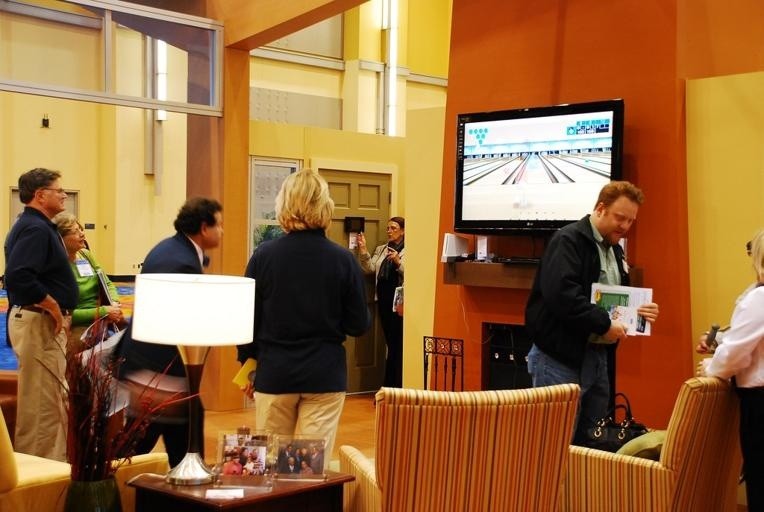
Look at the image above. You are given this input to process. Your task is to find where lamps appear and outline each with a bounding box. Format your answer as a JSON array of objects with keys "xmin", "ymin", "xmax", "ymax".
[{"xmin": 130, "ymin": 273, "xmax": 257, "ymax": 485}]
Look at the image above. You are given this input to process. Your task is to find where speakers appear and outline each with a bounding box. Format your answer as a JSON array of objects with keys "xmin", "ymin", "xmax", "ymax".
[{"xmin": 482, "ymin": 322, "xmax": 535, "ymax": 391}]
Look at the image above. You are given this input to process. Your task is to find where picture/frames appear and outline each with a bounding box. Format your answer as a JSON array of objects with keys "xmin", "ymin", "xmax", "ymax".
[
  {"xmin": 270, "ymin": 433, "xmax": 330, "ymax": 482},
  {"xmin": 215, "ymin": 431, "xmax": 272, "ymax": 484}
]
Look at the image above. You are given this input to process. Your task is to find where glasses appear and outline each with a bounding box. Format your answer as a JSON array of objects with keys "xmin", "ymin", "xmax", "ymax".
[{"xmin": 39, "ymin": 187, "xmax": 67, "ymax": 193}]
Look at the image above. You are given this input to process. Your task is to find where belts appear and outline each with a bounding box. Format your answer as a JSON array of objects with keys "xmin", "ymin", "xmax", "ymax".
[{"xmin": 12, "ymin": 303, "xmax": 74, "ymax": 317}]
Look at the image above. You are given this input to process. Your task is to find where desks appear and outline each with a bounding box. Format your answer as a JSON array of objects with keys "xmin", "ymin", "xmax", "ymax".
[{"xmin": 124, "ymin": 460, "xmax": 356, "ymax": 511}]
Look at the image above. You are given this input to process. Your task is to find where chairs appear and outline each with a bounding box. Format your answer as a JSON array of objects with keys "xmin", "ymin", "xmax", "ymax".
[
  {"xmin": 568, "ymin": 375, "xmax": 744, "ymax": 511},
  {"xmin": 1, "ymin": 403, "xmax": 168, "ymax": 512},
  {"xmin": 339, "ymin": 384, "xmax": 581, "ymax": 512}
]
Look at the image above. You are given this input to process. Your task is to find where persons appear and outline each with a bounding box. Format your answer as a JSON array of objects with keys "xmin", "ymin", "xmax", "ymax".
[
  {"xmin": 278, "ymin": 443, "xmax": 324, "ymax": 474},
  {"xmin": 223, "ymin": 427, "xmax": 268, "ymax": 476},
  {"xmin": 696, "ymin": 231, "xmax": 763, "ymax": 512},
  {"xmin": 3, "ymin": 168, "xmax": 122, "ymax": 463},
  {"xmin": 525, "ymin": 179, "xmax": 658, "ymax": 442},
  {"xmin": 357, "ymin": 217, "xmax": 405, "ymax": 388},
  {"xmin": 114, "ymin": 197, "xmax": 224, "ymax": 469},
  {"xmin": 234, "ymin": 167, "xmax": 373, "ymax": 456}
]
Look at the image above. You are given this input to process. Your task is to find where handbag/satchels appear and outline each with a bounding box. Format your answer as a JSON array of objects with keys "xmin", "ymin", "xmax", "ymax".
[{"xmin": 584, "ymin": 414, "xmax": 650, "ymax": 453}]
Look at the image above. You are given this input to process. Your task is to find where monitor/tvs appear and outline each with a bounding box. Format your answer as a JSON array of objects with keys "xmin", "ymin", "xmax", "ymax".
[{"xmin": 454, "ymin": 99, "xmax": 625, "ymax": 264}]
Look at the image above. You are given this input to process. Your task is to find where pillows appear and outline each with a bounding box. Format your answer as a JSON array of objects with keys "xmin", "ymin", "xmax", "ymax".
[{"xmin": 615, "ymin": 433, "xmax": 665, "ymax": 461}]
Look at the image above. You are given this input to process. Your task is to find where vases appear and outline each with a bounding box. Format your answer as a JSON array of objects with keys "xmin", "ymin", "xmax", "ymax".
[{"xmin": 62, "ymin": 475, "xmax": 123, "ymax": 512}]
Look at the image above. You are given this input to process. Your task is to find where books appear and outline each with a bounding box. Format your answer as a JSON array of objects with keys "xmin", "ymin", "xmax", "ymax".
[
  {"xmin": 590, "ymin": 282, "xmax": 653, "ymax": 337},
  {"xmin": 231, "ymin": 357, "xmax": 258, "ymax": 389},
  {"xmin": 79, "ymin": 313, "xmax": 118, "ymax": 349}
]
